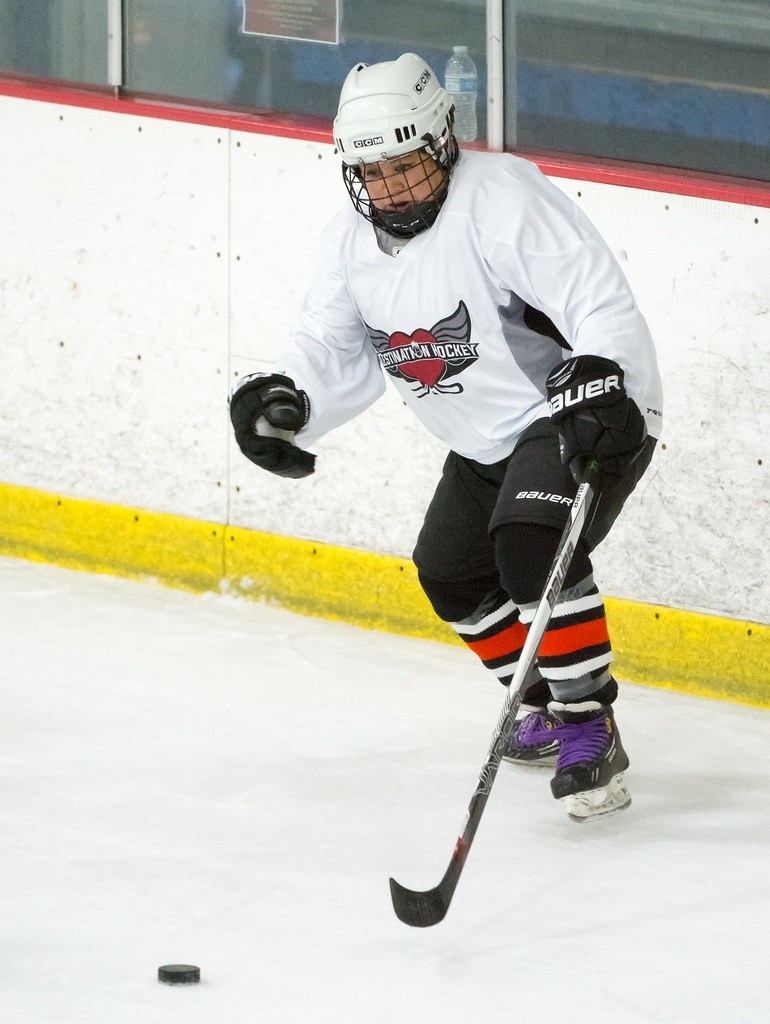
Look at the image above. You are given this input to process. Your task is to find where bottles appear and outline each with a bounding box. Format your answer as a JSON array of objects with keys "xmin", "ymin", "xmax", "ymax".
[{"xmin": 445, "ymin": 45, "xmax": 478, "ymax": 144}]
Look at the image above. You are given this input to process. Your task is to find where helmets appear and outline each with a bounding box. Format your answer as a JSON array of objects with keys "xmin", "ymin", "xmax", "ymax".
[{"xmin": 331, "ymin": 53, "xmax": 455, "ymax": 239}]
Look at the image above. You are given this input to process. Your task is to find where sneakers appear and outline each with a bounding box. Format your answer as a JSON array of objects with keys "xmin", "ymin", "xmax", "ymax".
[
  {"xmin": 500, "ymin": 710, "xmax": 564, "ymax": 767},
  {"xmin": 547, "ymin": 698, "xmax": 635, "ymax": 825}
]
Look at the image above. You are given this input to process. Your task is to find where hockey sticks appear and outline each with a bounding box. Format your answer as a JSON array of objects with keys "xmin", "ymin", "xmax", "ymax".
[{"xmin": 390, "ymin": 455, "xmax": 603, "ymax": 929}]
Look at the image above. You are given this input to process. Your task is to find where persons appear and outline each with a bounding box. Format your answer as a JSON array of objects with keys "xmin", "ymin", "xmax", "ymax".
[{"xmin": 228, "ymin": 52, "xmax": 662, "ymax": 825}]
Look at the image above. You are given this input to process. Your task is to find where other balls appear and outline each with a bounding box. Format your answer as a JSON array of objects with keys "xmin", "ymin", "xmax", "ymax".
[{"xmin": 158, "ymin": 963, "xmax": 201, "ymax": 985}]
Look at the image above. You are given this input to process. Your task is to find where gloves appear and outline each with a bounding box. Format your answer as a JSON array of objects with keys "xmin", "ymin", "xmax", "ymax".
[
  {"xmin": 545, "ymin": 355, "xmax": 648, "ymax": 490},
  {"xmin": 224, "ymin": 370, "xmax": 318, "ymax": 479}
]
